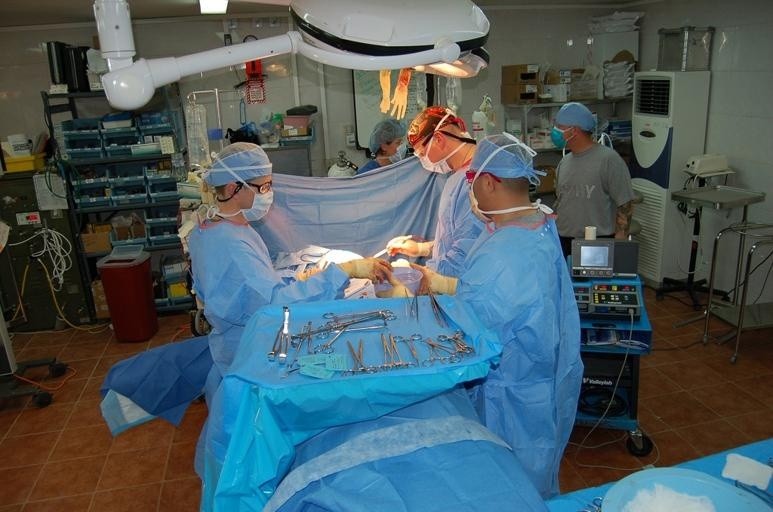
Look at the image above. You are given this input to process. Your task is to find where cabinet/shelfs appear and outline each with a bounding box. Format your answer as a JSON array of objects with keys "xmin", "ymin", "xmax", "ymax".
[
  {"xmin": 500, "ymin": 93, "xmax": 631, "ymax": 216},
  {"xmin": 36, "ymin": 73, "xmax": 205, "ymax": 325}
]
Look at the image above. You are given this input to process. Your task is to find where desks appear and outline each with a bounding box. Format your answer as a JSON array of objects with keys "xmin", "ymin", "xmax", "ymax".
[{"xmin": 541, "ymin": 426, "xmax": 772, "ymax": 511}]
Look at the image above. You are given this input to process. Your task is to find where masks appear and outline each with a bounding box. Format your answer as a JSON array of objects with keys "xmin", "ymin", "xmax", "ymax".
[
  {"xmin": 418, "ymin": 157, "xmax": 454, "ymax": 175},
  {"xmin": 467, "ymin": 189, "xmax": 492, "ymax": 225},
  {"xmin": 239, "ymin": 191, "xmax": 273, "ymax": 223},
  {"xmin": 388, "ymin": 143, "xmax": 408, "ymax": 166},
  {"xmin": 549, "ymin": 127, "xmax": 566, "ymax": 150}
]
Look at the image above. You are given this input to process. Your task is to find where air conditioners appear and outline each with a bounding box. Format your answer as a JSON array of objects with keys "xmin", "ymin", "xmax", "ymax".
[{"xmin": 623, "ymin": 69, "xmax": 712, "ymax": 296}]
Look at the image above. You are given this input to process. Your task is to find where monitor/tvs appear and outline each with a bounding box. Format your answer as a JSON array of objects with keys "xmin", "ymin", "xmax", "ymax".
[{"xmin": 263, "ymin": 145, "xmax": 313, "ymax": 178}]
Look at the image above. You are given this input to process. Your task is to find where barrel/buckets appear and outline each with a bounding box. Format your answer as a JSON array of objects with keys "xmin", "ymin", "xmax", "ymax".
[{"xmin": 472, "ymin": 109, "xmax": 489, "ymax": 140}]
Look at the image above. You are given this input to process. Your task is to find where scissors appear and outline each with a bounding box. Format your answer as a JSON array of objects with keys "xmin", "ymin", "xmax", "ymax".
[
  {"xmin": 287, "ymin": 310, "xmax": 475, "ymax": 376},
  {"xmin": 584, "ymin": 497, "xmax": 603, "ymax": 512}
]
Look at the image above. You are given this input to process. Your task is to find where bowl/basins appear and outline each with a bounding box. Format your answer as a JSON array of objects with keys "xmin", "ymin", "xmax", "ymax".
[{"xmin": 375, "ymin": 268, "xmax": 422, "ymax": 298}]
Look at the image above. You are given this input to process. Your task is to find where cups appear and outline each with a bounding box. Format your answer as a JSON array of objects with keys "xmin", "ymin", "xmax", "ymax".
[{"xmin": 583, "ymin": 225, "xmax": 595, "ymax": 240}]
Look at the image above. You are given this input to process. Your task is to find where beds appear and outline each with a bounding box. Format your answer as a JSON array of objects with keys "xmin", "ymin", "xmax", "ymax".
[{"xmin": 184, "ymin": 245, "xmax": 549, "ymax": 512}]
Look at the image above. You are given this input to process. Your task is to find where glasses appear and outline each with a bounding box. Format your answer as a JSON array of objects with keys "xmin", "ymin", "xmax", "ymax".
[
  {"xmin": 243, "ymin": 179, "xmax": 273, "ymax": 193},
  {"xmin": 412, "ymin": 134, "xmax": 436, "ymax": 157},
  {"xmin": 464, "ymin": 169, "xmax": 500, "ymax": 185}
]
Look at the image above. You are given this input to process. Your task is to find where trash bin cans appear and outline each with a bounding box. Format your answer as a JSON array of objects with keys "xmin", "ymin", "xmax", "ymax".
[{"xmin": 96, "ymin": 245, "xmax": 160, "ymax": 343}]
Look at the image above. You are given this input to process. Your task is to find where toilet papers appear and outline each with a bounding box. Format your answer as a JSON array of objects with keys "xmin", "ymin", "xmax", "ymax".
[{"xmin": 584, "ymin": 226, "xmax": 597, "ymax": 240}]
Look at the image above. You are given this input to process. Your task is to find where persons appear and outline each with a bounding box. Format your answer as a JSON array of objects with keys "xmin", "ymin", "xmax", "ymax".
[
  {"xmin": 369, "ymin": 131, "xmax": 585, "ymax": 500},
  {"xmin": 383, "ymin": 105, "xmax": 492, "ymax": 281},
  {"xmin": 548, "ymin": 102, "xmax": 636, "ymax": 261},
  {"xmin": 353, "ymin": 118, "xmax": 407, "ymax": 176},
  {"xmin": 188, "ymin": 138, "xmax": 394, "ymax": 378}
]
